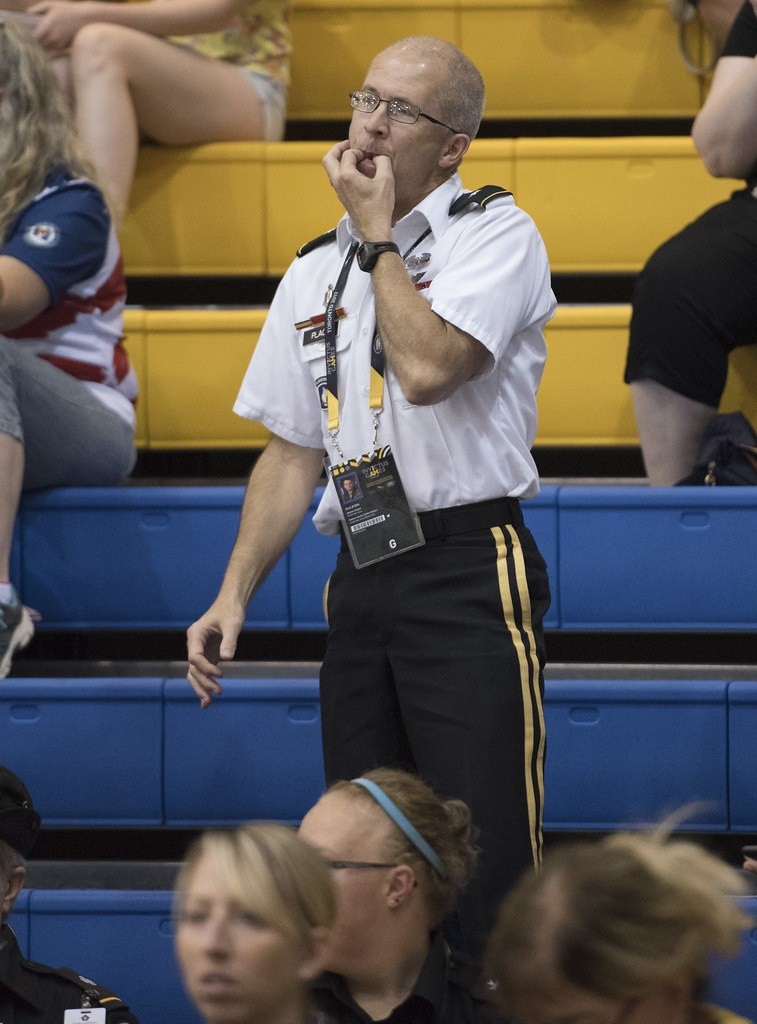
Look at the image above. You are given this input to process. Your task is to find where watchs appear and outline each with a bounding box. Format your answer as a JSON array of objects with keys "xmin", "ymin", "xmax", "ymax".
[{"xmin": 357, "ymin": 240, "xmax": 401, "ymax": 272}]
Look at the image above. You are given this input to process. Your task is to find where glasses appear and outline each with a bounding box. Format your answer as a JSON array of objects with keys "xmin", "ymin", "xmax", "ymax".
[{"xmin": 349, "ymin": 89, "xmax": 459, "ymax": 134}]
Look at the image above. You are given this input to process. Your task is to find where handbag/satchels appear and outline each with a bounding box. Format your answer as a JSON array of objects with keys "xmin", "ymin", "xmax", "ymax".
[{"xmin": 672, "ymin": 410, "xmax": 757, "ymax": 487}]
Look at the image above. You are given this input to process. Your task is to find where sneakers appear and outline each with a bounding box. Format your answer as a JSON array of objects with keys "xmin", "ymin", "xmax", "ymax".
[{"xmin": 0, "ymin": 601, "xmax": 34, "ymax": 679}]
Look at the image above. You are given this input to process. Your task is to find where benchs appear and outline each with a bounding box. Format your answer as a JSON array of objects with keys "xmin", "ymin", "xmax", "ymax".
[{"xmin": 1, "ymin": 0, "xmax": 756, "ymax": 1024}]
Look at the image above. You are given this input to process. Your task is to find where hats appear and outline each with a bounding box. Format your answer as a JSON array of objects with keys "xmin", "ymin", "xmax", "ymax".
[{"xmin": 0, "ymin": 765, "xmax": 41, "ymax": 862}]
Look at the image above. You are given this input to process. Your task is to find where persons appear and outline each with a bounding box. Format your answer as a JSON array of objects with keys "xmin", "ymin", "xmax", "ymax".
[
  {"xmin": 1, "ymin": 768, "xmax": 141, "ymax": 1024},
  {"xmin": 174, "ymin": 768, "xmax": 757, "ymax": 1024},
  {"xmin": 623, "ymin": 0, "xmax": 757, "ymax": 488},
  {"xmin": 27, "ymin": 1, "xmax": 289, "ymax": 225},
  {"xmin": 0, "ymin": 17, "xmax": 134, "ymax": 678},
  {"xmin": 188, "ymin": 34, "xmax": 550, "ymax": 960}
]
